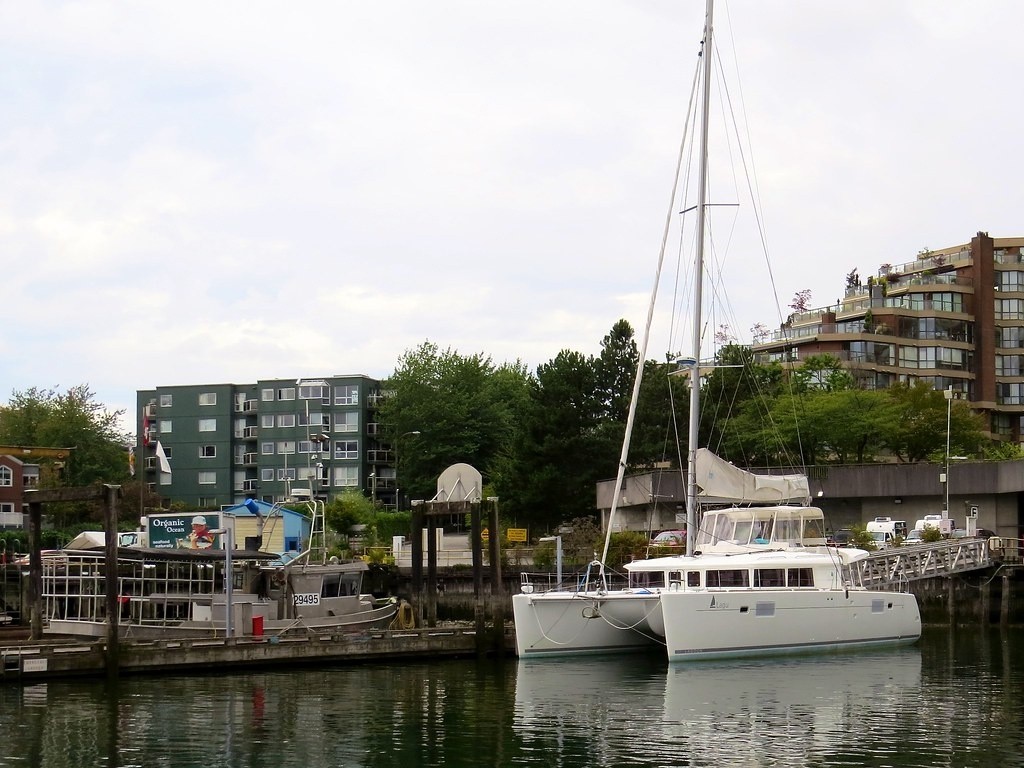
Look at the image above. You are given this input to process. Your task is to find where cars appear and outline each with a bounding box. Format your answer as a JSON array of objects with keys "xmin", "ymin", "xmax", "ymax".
[
  {"xmin": 653, "ymin": 530, "xmax": 686, "ymax": 552},
  {"xmin": 857, "ymin": 530, "xmax": 894, "ymax": 552},
  {"xmin": 832, "ymin": 528, "xmax": 859, "ymax": 550},
  {"xmin": 900, "ymin": 528, "xmax": 926, "ymax": 546}
]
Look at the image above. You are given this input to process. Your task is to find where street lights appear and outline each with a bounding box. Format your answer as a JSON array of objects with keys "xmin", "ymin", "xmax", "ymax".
[
  {"xmin": 395, "ymin": 431, "xmax": 421, "ymax": 489},
  {"xmin": 209, "ymin": 528, "xmax": 235, "ymax": 638}
]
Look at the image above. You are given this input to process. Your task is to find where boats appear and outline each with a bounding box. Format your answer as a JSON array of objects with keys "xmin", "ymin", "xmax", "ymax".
[{"xmin": 12, "ymin": 547, "xmax": 402, "ymax": 651}]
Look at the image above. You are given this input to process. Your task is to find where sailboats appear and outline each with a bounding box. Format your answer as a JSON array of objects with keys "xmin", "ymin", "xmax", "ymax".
[{"xmin": 510, "ymin": 1, "xmax": 927, "ymax": 663}]
